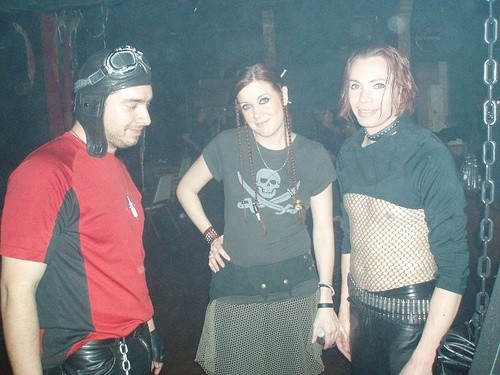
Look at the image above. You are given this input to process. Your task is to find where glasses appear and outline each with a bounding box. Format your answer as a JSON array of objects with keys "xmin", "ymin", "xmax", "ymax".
[{"xmin": 103, "ymin": 46, "xmax": 152, "ymax": 77}]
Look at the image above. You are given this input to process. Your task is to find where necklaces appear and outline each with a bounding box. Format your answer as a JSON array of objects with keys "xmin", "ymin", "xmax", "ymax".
[
  {"xmin": 71, "ymin": 129, "xmax": 138, "ymax": 221},
  {"xmin": 253, "ymin": 131, "xmax": 290, "ymax": 172}
]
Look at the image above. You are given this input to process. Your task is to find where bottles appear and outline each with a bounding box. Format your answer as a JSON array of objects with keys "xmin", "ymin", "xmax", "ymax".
[{"xmin": 319, "ymin": 331, "xmax": 338, "ymax": 350}]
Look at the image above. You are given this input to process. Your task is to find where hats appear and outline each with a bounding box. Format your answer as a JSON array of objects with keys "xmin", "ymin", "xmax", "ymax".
[{"xmin": 76, "ymin": 49, "xmax": 152, "ymax": 158}]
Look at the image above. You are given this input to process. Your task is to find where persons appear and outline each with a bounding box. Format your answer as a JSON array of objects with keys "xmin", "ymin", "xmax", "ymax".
[
  {"xmin": 335, "ymin": 45, "xmax": 470, "ymax": 375},
  {"xmin": 0, "ymin": 45, "xmax": 165, "ymax": 375},
  {"xmin": 175, "ymin": 63, "xmax": 348, "ymax": 375}
]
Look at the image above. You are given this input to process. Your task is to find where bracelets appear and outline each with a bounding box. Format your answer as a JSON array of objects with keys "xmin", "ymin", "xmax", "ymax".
[
  {"xmin": 202, "ymin": 226, "xmax": 219, "ymax": 245},
  {"xmin": 317, "ymin": 282, "xmax": 335, "ymax": 296},
  {"xmin": 317, "ymin": 302, "xmax": 335, "ymax": 309}
]
